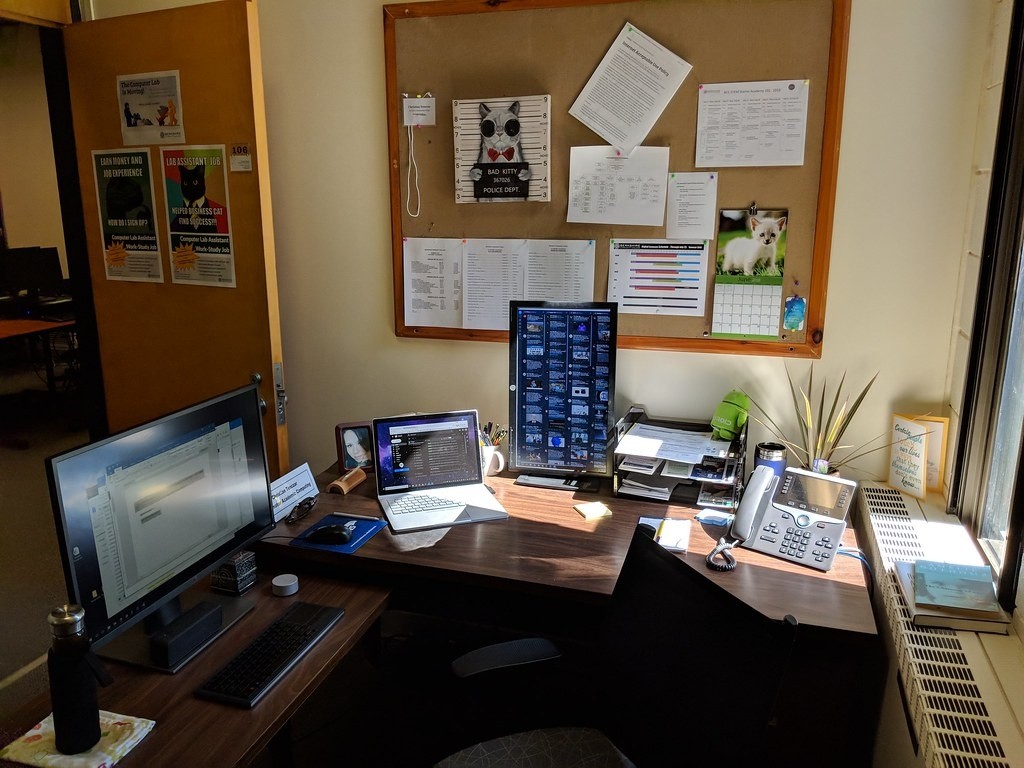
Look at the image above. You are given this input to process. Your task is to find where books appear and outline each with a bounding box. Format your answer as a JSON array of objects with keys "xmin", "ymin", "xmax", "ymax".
[
  {"xmin": 618, "ymin": 472, "xmax": 679, "ymax": 500},
  {"xmin": 574, "ymin": 501, "xmax": 612, "ymax": 519},
  {"xmin": 638, "ymin": 517, "xmax": 691, "ymax": 554},
  {"xmin": 893, "ymin": 560, "xmax": 1012, "ymax": 635}
]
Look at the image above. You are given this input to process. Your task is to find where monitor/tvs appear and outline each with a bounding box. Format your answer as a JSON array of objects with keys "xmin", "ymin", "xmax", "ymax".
[{"xmin": 45, "ymin": 383, "xmax": 277, "ymax": 674}]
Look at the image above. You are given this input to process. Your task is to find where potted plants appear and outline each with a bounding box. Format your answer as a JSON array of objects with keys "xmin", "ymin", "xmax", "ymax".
[{"xmin": 736, "ymin": 360, "xmax": 936, "ymax": 479}]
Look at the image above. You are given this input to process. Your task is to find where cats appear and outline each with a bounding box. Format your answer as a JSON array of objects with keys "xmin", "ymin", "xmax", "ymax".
[
  {"xmin": 722, "ymin": 217, "xmax": 785, "ymax": 276},
  {"xmin": 169, "ymin": 159, "xmax": 228, "ymax": 233},
  {"xmin": 469, "ymin": 101, "xmax": 532, "ymax": 202}
]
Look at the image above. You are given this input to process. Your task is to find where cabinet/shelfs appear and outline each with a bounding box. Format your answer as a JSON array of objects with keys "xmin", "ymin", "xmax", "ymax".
[{"xmin": 606, "ymin": 406, "xmax": 749, "ymax": 514}]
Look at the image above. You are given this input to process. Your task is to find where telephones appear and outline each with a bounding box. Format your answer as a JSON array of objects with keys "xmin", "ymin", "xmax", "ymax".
[{"xmin": 730, "ymin": 464, "xmax": 857, "ymax": 571}]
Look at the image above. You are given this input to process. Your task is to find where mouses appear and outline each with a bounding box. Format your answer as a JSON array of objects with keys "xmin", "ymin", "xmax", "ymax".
[{"xmin": 304, "ymin": 524, "xmax": 352, "ymax": 545}]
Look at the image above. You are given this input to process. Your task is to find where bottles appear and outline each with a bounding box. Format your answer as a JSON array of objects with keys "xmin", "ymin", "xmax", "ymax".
[{"xmin": 47, "ymin": 604, "xmax": 102, "ymax": 754}]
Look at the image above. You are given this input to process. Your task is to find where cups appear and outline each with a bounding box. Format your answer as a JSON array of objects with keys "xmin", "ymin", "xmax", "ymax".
[
  {"xmin": 755, "ymin": 442, "xmax": 787, "ymax": 480},
  {"xmin": 482, "ymin": 444, "xmax": 505, "ymax": 476}
]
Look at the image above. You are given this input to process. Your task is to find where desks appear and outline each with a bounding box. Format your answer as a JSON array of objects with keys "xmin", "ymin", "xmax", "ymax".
[{"xmin": 0, "ymin": 319, "xmax": 873, "ymax": 768}]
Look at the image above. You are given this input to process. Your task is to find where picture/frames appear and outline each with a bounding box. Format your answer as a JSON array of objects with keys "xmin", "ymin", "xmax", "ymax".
[{"xmin": 335, "ymin": 420, "xmax": 375, "ymax": 472}]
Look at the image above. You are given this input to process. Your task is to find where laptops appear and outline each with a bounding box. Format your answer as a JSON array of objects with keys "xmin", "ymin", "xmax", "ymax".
[{"xmin": 372, "ymin": 410, "xmax": 508, "ymax": 533}]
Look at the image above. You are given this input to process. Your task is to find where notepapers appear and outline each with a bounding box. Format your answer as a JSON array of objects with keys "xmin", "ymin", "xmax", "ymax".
[{"xmin": 574, "ymin": 501, "xmax": 612, "ymax": 519}]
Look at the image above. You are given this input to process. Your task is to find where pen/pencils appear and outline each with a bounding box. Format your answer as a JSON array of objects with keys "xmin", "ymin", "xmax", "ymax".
[
  {"xmin": 479, "ymin": 421, "xmax": 508, "ymax": 446},
  {"xmin": 332, "ymin": 511, "xmax": 380, "ymax": 520},
  {"xmin": 655, "ymin": 520, "xmax": 667, "ymax": 543}
]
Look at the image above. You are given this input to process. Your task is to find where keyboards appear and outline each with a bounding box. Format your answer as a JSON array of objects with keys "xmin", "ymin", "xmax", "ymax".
[{"xmin": 192, "ymin": 601, "xmax": 345, "ymax": 710}]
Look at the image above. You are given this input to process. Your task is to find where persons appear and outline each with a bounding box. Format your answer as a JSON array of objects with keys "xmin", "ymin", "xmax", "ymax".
[{"xmin": 344, "ymin": 429, "xmax": 372, "ymax": 469}]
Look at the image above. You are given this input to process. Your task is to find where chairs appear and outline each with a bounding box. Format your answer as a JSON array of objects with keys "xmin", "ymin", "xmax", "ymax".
[{"xmin": 429, "ymin": 636, "xmax": 637, "ymax": 768}]
